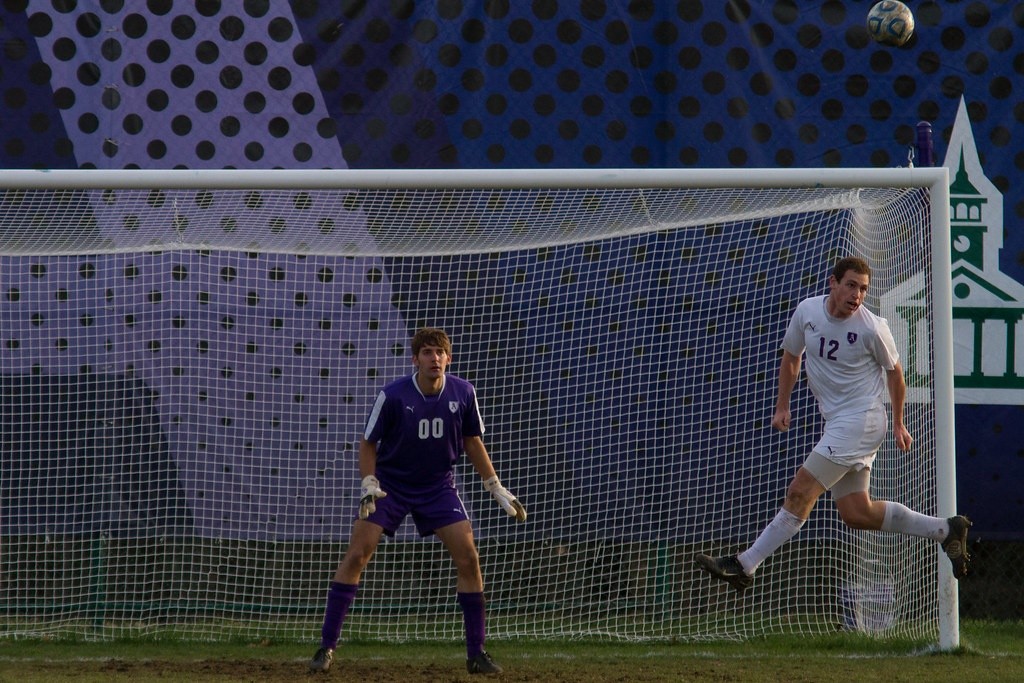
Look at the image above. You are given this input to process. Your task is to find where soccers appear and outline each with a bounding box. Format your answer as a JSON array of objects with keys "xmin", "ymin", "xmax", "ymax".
[{"xmin": 865, "ymin": 0, "xmax": 914, "ymax": 49}]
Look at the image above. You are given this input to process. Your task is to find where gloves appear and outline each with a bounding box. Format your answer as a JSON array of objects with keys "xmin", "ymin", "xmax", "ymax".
[
  {"xmin": 359, "ymin": 475, "xmax": 387, "ymax": 519},
  {"xmin": 484, "ymin": 475, "xmax": 526, "ymax": 521}
]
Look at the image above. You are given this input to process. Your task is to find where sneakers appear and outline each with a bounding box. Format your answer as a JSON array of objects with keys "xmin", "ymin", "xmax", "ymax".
[
  {"xmin": 310, "ymin": 647, "xmax": 333, "ymax": 671},
  {"xmin": 695, "ymin": 553, "xmax": 754, "ymax": 591},
  {"xmin": 941, "ymin": 515, "xmax": 972, "ymax": 580},
  {"xmin": 466, "ymin": 651, "xmax": 503, "ymax": 674}
]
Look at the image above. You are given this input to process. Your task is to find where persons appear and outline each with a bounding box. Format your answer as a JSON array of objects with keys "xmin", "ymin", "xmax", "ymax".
[
  {"xmin": 308, "ymin": 328, "xmax": 526, "ymax": 675},
  {"xmin": 695, "ymin": 257, "xmax": 972, "ymax": 592}
]
